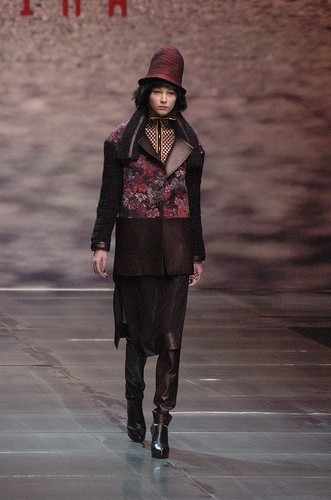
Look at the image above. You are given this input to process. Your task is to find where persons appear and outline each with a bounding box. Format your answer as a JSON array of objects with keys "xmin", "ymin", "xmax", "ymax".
[{"xmin": 91, "ymin": 45, "xmax": 206, "ymax": 458}]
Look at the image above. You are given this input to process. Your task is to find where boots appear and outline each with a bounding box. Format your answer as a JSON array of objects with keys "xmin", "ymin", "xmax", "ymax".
[
  {"xmin": 126, "ymin": 398, "xmax": 146, "ymax": 443},
  {"xmin": 149, "ymin": 408, "xmax": 172, "ymax": 459}
]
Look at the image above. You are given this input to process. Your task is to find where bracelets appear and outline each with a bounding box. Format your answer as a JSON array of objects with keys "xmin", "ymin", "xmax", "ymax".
[
  {"xmin": 194, "ymin": 254, "xmax": 205, "ymax": 261},
  {"xmin": 91, "ymin": 241, "xmax": 110, "ymax": 252}
]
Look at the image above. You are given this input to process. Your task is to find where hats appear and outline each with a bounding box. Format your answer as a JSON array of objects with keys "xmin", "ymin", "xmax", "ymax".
[{"xmin": 138, "ymin": 45, "xmax": 188, "ymax": 94}]
